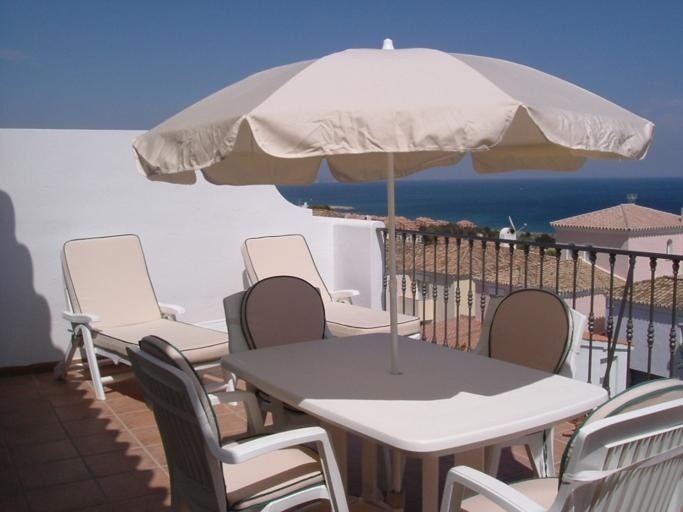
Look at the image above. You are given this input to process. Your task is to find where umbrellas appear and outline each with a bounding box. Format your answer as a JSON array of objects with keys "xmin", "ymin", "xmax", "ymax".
[{"xmin": 129, "ymin": 40, "xmax": 655, "ymax": 495}]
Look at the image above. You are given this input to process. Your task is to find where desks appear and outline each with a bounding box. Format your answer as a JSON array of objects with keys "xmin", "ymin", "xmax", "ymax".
[{"xmin": 218, "ymin": 331, "xmax": 611, "ymax": 512}]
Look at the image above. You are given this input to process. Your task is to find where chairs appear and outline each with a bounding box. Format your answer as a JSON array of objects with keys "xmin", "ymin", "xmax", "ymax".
[
  {"xmin": 437, "ymin": 375, "xmax": 683, "ymax": 511},
  {"xmin": 56, "ymin": 234, "xmax": 237, "ymax": 405},
  {"xmin": 427, "ymin": 287, "xmax": 588, "ymax": 481},
  {"xmin": 242, "ymin": 235, "xmax": 424, "ymax": 345},
  {"xmin": 240, "ymin": 274, "xmax": 343, "ymax": 436},
  {"xmin": 124, "ymin": 333, "xmax": 352, "ymax": 511}
]
